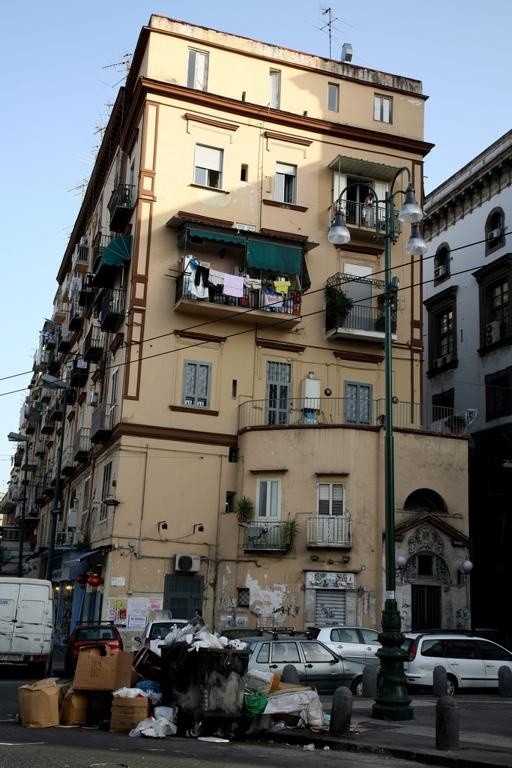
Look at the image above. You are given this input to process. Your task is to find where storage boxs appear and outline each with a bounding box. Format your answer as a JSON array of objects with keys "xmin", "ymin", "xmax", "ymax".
[{"xmin": 18, "ymin": 643, "xmax": 151, "ymax": 734}]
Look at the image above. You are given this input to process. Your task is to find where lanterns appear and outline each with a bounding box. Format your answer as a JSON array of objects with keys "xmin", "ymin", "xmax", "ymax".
[
  {"xmin": 87, "ymin": 572, "xmax": 103, "ymax": 592},
  {"xmin": 76, "ymin": 575, "xmax": 87, "ymax": 584}
]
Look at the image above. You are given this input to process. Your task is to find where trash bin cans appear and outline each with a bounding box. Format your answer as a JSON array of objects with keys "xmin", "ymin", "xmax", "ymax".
[{"xmin": 158, "ymin": 644, "xmax": 254, "ymax": 739}]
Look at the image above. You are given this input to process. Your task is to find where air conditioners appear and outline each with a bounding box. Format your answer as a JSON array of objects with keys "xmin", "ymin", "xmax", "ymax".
[
  {"xmin": 485, "ymin": 227, "xmax": 502, "ymax": 244},
  {"xmin": 484, "ymin": 319, "xmax": 501, "ymax": 346},
  {"xmin": 432, "ymin": 265, "xmax": 449, "ymax": 278},
  {"xmin": 173, "ymin": 553, "xmax": 202, "ymax": 574},
  {"xmin": 430, "ymin": 353, "xmax": 454, "ymax": 370}
]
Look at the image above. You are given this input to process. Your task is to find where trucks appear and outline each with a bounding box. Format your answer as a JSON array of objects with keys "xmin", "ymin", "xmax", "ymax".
[{"xmin": 0, "ymin": 576, "xmax": 57, "ymax": 680}]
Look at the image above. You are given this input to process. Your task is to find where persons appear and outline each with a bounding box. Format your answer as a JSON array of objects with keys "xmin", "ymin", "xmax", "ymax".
[
  {"xmin": 362, "ymin": 192, "xmax": 375, "ymax": 226},
  {"xmin": 192, "ymin": 609, "xmax": 205, "ymax": 628}
]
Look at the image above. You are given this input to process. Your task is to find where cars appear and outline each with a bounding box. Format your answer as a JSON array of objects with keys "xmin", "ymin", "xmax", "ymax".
[
  {"xmin": 305, "ymin": 624, "xmax": 384, "ymax": 675},
  {"xmin": 134, "ymin": 618, "xmax": 189, "ymax": 658}
]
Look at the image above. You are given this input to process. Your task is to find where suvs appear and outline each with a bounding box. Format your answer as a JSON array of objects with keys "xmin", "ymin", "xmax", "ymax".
[
  {"xmin": 63, "ymin": 619, "xmax": 124, "ymax": 675},
  {"xmin": 237, "ymin": 626, "xmax": 381, "ymax": 697}
]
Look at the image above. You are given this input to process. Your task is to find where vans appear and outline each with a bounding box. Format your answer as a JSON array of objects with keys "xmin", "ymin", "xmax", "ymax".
[{"xmin": 401, "ymin": 630, "xmax": 512, "ymax": 698}]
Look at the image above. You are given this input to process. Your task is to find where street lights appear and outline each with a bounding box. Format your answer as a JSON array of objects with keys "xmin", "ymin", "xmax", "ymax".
[{"xmin": 325, "ymin": 165, "xmax": 430, "ymax": 722}]
